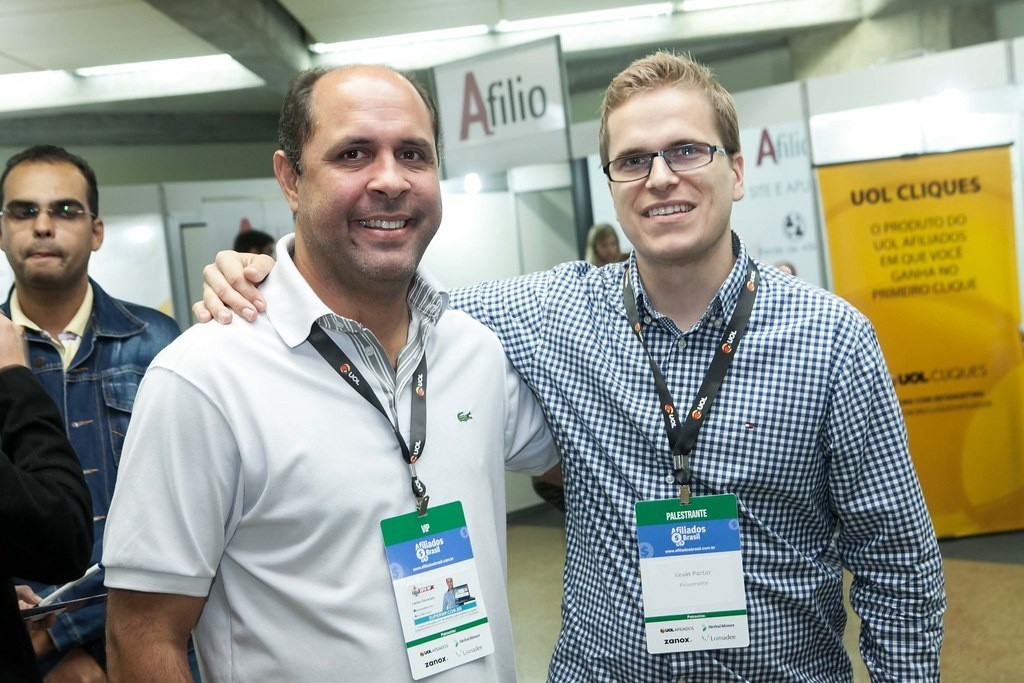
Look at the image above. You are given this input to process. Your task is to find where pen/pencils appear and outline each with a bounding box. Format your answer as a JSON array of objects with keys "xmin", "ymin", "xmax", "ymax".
[{"xmin": 31, "ymin": 562, "xmax": 105, "ymax": 607}]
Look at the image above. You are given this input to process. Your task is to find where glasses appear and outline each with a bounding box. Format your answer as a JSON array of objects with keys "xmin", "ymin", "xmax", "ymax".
[
  {"xmin": 603, "ymin": 142, "xmax": 735, "ymax": 183},
  {"xmin": 0, "ymin": 203, "xmax": 98, "ymax": 219}
]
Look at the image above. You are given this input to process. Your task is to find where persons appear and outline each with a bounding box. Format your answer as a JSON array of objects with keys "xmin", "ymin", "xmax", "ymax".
[
  {"xmin": 586, "ymin": 224, "xmax": 632, "ymax": 269},
  {"xmin": 193, "ymin": 52, "xmax": 947, "ymax": 683},
  {"xmin": 443, "ymin": 578, "xmax": 463, "ymax": 611},
  {"xmin": 100, "ymin": 61, "xmax": 563, "ymax": 683},
  {"xmin": 234, "ymin": 230, "xmax": 275, "ymax": 261},
  {"xmin": 0, "ymin": 145, "xmax": 183, "ymax": 683}
]
